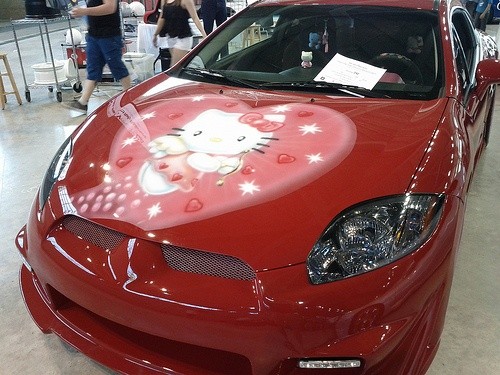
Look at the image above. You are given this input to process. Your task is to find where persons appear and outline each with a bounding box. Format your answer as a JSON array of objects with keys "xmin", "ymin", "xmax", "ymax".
[
  {"xmin": 152, "ymin": 0, "xmax": 207, "ymax": 68},
  {"xmin": 198, "ymin": 0, "xmax": 229, "ymax": 60},
  {"xmin": 61, "ymin": 0, "xmax": 131, "ymax": 114},
  {"xmin": 474, "ymin": 0, "xmax": 493, "ymax": 32}
]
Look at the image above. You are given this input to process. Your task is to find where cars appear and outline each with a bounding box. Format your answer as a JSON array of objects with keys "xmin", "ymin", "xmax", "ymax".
[{"xmin": 15, "ymin": 0, "xmax": 500, "ymax": 375}]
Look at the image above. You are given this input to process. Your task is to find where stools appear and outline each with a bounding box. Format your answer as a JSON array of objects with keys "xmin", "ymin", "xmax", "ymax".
[
  {"xmin": 0, "ymin": 51, "xmax": 23, "ymax": 110},
  {"xmin": 242, "ymin": 24, "xmax": 262, "ymax": 48}
]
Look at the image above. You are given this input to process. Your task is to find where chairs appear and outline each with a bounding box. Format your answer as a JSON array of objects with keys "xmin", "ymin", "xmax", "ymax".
[
  {"xmin": 371, "ymin": 22, "xmax": 430, "ymax": 80},
  {"xmin": 282, "ymin": 29, "xmax": 331, "ymax": 70},
  {"xmin": 254, "ymin": 15, "xmax": 274, "ymax": 39}
]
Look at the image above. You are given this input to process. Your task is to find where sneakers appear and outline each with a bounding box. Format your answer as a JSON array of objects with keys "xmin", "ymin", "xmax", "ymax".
[{"xmin": 61, "ymin": 101, "xmax": 87, "ymax": 113}]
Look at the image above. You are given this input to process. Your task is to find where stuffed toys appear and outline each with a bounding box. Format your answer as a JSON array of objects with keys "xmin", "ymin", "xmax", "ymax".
[
  {"xmin": 301, "ymin": 51, "xmax": 312, "ymax": 68},
  {"xmin": 309, "ymin": 32, "xmax": 321, "ymax": 50},
  {"xmin": 407, "ymin": 36, "xmax": 424, "ymax": 53}
]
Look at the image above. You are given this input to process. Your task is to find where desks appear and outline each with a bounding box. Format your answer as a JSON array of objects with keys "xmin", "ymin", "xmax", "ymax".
[{"xmin": 136, "ymin": 20, "xmax": 217, "ymax": 54}]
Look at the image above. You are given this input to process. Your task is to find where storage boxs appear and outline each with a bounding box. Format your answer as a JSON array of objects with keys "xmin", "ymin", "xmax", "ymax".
[{"xmin": 121, "ymin": 54, "xmax": 155, "ymax": 84}]
